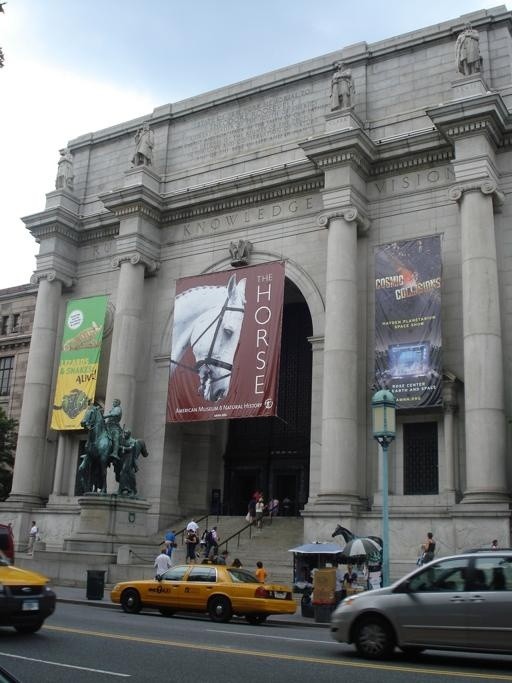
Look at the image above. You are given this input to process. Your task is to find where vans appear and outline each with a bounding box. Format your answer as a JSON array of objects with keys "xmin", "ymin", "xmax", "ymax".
[{"xmin": 0, "ymin": 524, "xmax": 16, "ymax": 565}]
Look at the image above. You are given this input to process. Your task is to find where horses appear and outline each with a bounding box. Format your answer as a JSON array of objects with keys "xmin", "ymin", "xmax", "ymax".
[
  {"xmin": 331, "ymin": 524, "xmax": 383, "ymax": 549},
  {"xmin": 78, "ymin": 403, "xmax": 149, "ymax": 493},
  {"xmin": 170, "ymin": 272, "xmax": 247, "ymax": 403}
]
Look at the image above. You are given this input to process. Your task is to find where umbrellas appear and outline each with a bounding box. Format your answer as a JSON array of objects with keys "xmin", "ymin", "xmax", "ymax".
[{"xmin": 342, "ymin": 537, "xmax": 383, "ymax": 571}]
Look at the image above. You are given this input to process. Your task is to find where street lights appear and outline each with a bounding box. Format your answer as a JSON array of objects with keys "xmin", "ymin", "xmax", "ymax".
[{"xmin": 373, "ymin": 390, "xmax": 398, "ymax": 588}]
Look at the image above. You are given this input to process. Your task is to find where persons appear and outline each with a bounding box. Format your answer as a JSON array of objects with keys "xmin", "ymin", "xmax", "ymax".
[
  {"xmin": 491, "ymin": 539, "xmax": 498, "ymax": 549},
  {"xmin": 330, "ymin": 559, "xmax": 345, "ymax": 603},
  {"xmin": 454, "ymin": 20, "xmax": 485, "ymax": 76},
  {"xmin": 55, "ymin": 147, "xmax": 76, "ymax": 192},
  {"xmin": 255, "ymin": 561, "xmax": 268, "ymax": 583},
  {"xmin": 117, "ymin": 428, "xmax": 138, "ymax": 495},
  {"xmin": 343, "ymin": 564, "xmax": 357, "ymax": 582},
  {"xmin": 28, "ymin": 520, "xmax": 39, "ymax": 555},
  {"xmin": 244, "ymin": 487, "xmax": 291, "ymax": 529},
  {"xmin": 154, "ymin": 517, "xmax": 244, "ymax": 577},
  {"xmin": 100, "ymin": 398, "xmax": 122, "ymax": 460},
  {"xmin": 8, "ymin": 521, "xmax": 15, "ymax": 542},
  {"xmin": 422, "ymin": 531, "xmax": 436, "ymax": 564},
  {"xmin": 129, "ymin": 120, "xmax": 157, "ymax": 167},
  {"xmin": 329, "ymin": 60, "xmax": 357, "ymax": 110}
]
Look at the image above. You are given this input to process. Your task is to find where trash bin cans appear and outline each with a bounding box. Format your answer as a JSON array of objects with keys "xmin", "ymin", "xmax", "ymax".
[{"xmin": 86, "ymin": 569, "xmax": 107, "ymax": 600}]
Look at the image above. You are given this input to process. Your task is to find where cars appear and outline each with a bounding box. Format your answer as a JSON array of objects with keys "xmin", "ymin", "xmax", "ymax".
[
  {"xmin": 0, "ymin": 550, "xmax": 56, "ymax": 634},
  {"xmin": 111, "ymin": 564, "xmax": 298, "ymax": 624},
  {"xmin": 0, "ymin": 663, "xmax": 21, "ymax": 683},
  {"xmin": 330, "ymin": 548, "xmax": 512, "ymax": 659}
]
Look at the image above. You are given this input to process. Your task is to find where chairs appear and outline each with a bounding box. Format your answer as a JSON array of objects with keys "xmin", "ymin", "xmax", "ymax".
[
  {"xmin": 472, "ymin": 570, "xmax": 489, "ymax": 591},
  {"xmin": 492, "ymin": 567, "xmax": 506, "ymax": 591}
]
